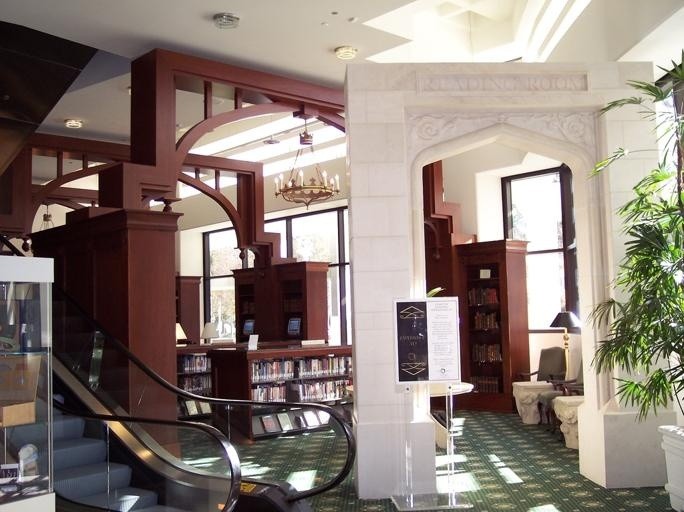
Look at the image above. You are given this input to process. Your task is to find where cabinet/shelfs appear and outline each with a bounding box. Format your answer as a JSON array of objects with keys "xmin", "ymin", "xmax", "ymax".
[
  {"xmin": 169, "ymin": 259, "xmax": 356, "ymax": 444},
  {"xmin": 449, "ymin": 236, "xmax": 530, "ymax": 414}
]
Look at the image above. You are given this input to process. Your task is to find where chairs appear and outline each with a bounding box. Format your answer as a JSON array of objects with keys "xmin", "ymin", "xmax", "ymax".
[{"xmin": 511, "ymin": 346, "xmax": 583, "ymax": 433}]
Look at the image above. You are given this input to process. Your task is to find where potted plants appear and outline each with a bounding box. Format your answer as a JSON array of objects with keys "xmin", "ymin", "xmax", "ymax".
[{"xmin": 582, "ymin": 56, "xmax": 684, "ymax": 512}]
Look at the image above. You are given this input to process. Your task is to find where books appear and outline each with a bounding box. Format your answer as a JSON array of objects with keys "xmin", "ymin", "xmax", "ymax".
[
  {"xmin": 178, "ymin": 354, "xmax": 354, "ymax": 433},
  {"xmin": 283, "ymin": 299, "xmax": 303, "ymax": 314},
  {"xmin": 241, "ymin": 301, "xmax": 255, "ymax": 315},
  {"xmin": 467, "ymin": 287, "xmax": 502, "ymax": 394}
]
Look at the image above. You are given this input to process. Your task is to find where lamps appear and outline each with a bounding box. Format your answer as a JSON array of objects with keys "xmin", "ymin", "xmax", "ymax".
[
  {"xmin": 271, "ymin": 112, "xmax": 343, "ymax": 210},
  {"xmin": 549, "ymin": 310, "xmax": 581, "ymax": 379}
]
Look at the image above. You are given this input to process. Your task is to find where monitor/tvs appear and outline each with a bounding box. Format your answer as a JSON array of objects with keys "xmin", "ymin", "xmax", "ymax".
[
  {"xmin": 242, "ymin": 320, "xmax": 266, "ymax": 341},
  {"xmin": 288, "ymin": 317, "xmax": 302, "ymax": 336}
]
